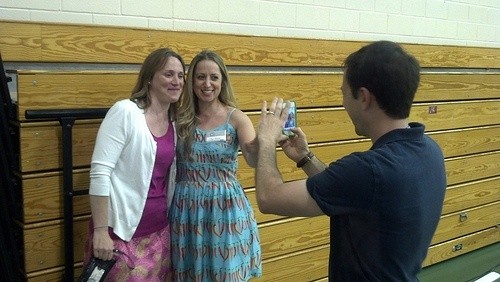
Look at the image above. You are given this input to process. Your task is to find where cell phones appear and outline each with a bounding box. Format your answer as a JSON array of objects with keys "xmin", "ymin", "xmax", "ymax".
[{"xmin": 281, "ymin": 100, "xmax": 298, "ymax": 136}]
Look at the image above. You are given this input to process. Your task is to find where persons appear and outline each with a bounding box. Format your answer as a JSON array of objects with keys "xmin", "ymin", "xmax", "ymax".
[
  {"xmin": 286, "ymin": 115, "xmax": 294, "ymax": 127},
  {"xmin": 255, "ymin": 41, "xmax": 446, "ymax": 282},
  {"xmin": 84, "ymin": 48, "xmax": 188, "ymax": 282},
  {"xmin": 169, "ymin": 51, "xmax": 262, "ymax": 282}
]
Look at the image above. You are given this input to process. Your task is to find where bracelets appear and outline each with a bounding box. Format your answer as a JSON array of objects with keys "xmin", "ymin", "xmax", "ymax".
[{"xmin": 297, "ymin": 152, "xmax": 314, "ymax": 168}]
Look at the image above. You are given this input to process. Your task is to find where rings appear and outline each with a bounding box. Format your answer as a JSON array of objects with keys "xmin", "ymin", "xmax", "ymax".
[{"xmin": 269, "ymin": 112, "xmax": 274, "ymax": 115}]
